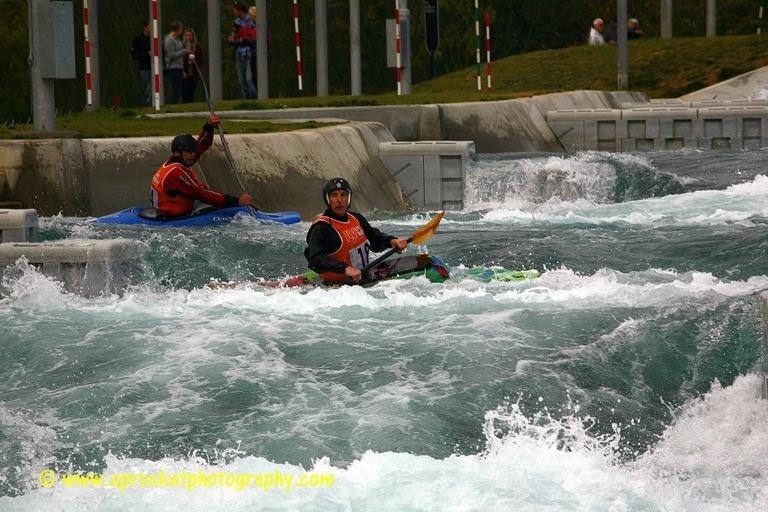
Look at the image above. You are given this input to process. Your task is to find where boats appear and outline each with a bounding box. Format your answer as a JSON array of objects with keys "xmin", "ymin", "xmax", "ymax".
[
  {"xmin": 76, "ymin": 203, "xmax": 302, "ymax": 229},
  {"xmin": 202, "ymin": 257, "xmax": 542, "ymax": 293}
]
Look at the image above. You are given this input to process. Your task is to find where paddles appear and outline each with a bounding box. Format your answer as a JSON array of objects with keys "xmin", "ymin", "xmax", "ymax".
[{"xmin": 361, "ymin": 211, "xmax": 445, "ymax": 276}]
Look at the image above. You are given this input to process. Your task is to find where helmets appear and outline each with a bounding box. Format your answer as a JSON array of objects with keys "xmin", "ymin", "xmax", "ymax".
[
  {"xmin": 321, "ymin": 178, "xmax": 355, "ymax": 210},
  {"xmin": 170, "ymin": 133, "xmax": 199, "ymax": 152}
]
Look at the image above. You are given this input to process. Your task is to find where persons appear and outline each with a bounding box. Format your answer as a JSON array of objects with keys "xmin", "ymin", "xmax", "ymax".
[
  {"xmin": 226, "ymin": 1, "xmax": 273, "ymax": 99},
  {"xmin": 162, "ymin": 20, "xmax": 192, "ymax": 105},
  {"xmin": 627, "ymin": 18, "xmax": 646, "ymax": 39},
  {"xmin": 148, "ymin": 109, "xmax": 253, "ymax": 219},
  {"xmin": 181, "ymin": 27, "xmax": 203, "ymax": 103},
  {"xmin": 130, "ymin": 20, "xmax": 151, "ymax": 107},
  {"xmin": 587, "ymin": 18, "xmax": 606, "ymax": 45},
  {"xmin": 303, "ymin": 178, "xmax": 408, "ymax": 286}
]
